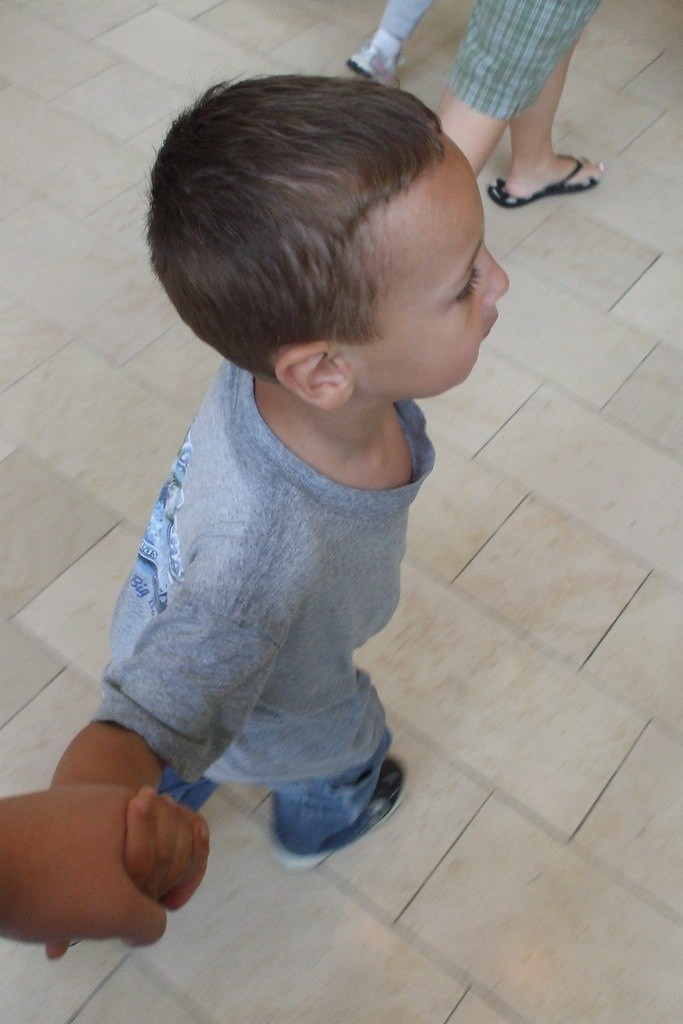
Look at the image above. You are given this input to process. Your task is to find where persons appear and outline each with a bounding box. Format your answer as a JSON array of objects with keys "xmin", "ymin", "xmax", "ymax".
[
  {"xmin": 1, "ymin": 789, "xmax": 167, "ymax": 949},
  {"xmin": 46, "ymin": 76, "xmax": 513, "ymax": 963},
  {"xmin": 348, "ymin": 0, "xmax": 604, "ymax": 208}
]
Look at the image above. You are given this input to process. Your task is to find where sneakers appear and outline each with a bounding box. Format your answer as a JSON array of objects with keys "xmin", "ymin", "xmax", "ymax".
[
  {"xmin": 264, "ymin": 757, "xmax": 405, "ymax": 868},
  {"xmin": 346, "ymin": 41, "xmax": 401, "ymax": 87}
]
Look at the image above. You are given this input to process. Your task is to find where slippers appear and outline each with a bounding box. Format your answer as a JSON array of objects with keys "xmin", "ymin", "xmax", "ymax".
[{"xmin": 488, "ymin": 156, "xmax": 598, "ymax": 208}]
